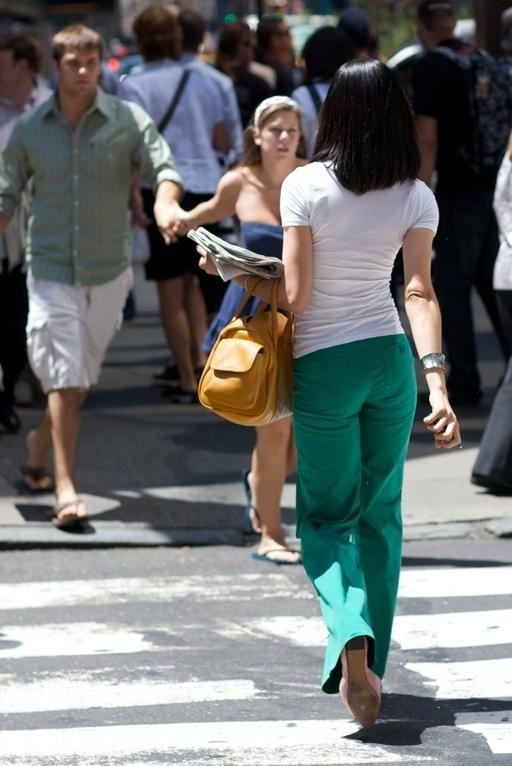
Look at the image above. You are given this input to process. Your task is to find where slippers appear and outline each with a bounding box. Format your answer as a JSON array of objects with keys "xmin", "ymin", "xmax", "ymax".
[
  {"xmin": 239, "ymin": 463, "xmax": 263, "ymax": 536},
  {"xmin": 251, "ymin": 545, "xmax": 307, "ymax": 567},
  {"xmin": 16, "ymin": 457, "xmax": 55, "ymax": 494},
  {"xmin": 43, "ymin": 496, "xmax": 95, "ymax": 531}
]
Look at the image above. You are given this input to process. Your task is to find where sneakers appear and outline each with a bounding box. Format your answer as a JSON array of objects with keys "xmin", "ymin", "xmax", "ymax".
[{"xmin": 158, "ymin": 382, "xmax": 196, "ymax": 406}]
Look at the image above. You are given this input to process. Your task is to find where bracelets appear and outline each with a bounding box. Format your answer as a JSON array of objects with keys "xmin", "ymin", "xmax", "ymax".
[
  {"xmin": 244, "ymin": 276, "xmax": 254, "ymax": 297},
  {"xmin": 421, "ymin": 351, "xmax": 451, "ymax": 376}
]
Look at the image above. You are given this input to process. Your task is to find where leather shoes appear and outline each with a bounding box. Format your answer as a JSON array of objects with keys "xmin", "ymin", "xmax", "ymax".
[{"xmin": 337, "ymin": 633, "xmax": 384, "ymax": 731}]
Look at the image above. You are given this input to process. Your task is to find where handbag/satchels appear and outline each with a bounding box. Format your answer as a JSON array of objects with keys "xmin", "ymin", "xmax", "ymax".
[{"xmin": 196, "ymin": 274, "xmax": 293, "ymax": 429}]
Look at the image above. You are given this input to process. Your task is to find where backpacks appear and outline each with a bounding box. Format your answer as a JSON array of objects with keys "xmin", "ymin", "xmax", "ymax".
[{"xmin": 432, "ymin": 45, "xmax": 511, "ymax": 165}]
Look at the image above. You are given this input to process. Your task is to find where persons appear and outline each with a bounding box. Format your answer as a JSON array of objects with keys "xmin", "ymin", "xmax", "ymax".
[
  {"xmin": 252, "ymin": 10, "xmax": 302, "ymax": 96},
  {"xmin": 335, "ymin": 10, "xmax": 386, "ymax": 61},
  {"xmin": 174, "ymin": 96, "xmax": 315, "ymax": 564},
  {"xmin": 196, "ymin": 57, "xmax": 462, "ymax": 730},
  {"xmin": 0, "ymin": 25, "xmax": 185, "ymax": 527},
  {"xmin": 290, "ymin": 26, "xmax": 354, "ymax": 160},
  {"xmin": 206, "ymin": 21, "xmax": 275, "ymax": 131},
  {"xmin": 0, "ymin": 33, "xmax": 53, "ymax": 431},
  {"xmin": 395, "ymin": 0, "xmax": 511, "ymax": 409},
  {"xmin": 470, "ymin": 125, "xmax": 512, "ymax": 500},
  {"xmin": 113, "ymin": 7, "xmax": 230, "ymax": 406},
  {"xmin": 177, "ymin": 10, "xmax": 245, "ymax": 324}
]
